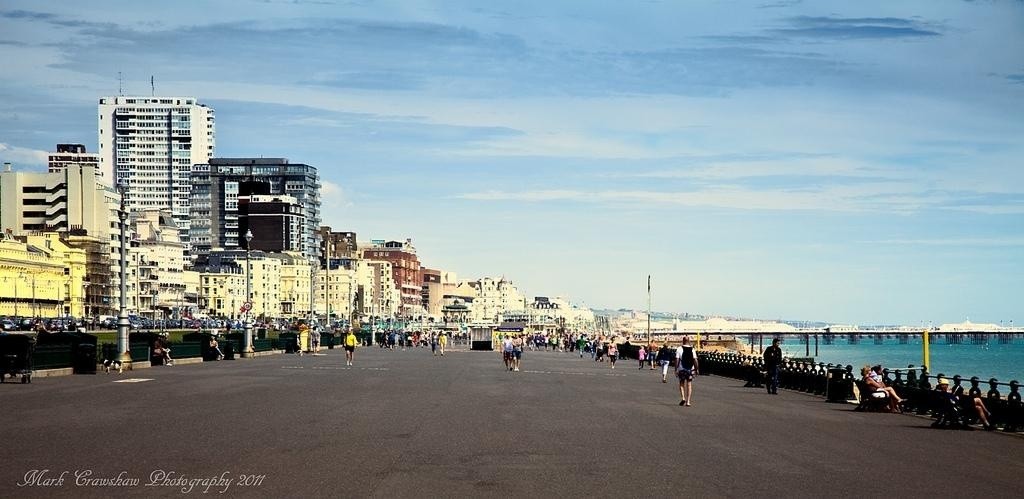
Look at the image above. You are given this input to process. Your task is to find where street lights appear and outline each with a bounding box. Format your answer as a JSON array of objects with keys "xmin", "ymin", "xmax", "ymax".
[
  {"xmin": 371, "ymin": 282, "xmax": 378, "ymax": 345},
  {"xmin": 307, "ymin": 255, "xmax": 316, "ymax": 333},
  {"xmin": 347, "ymin": 271, "xmax": 355, "ymax": 332},
  {"xmin": 412, "ymin": 297, "xmax": 417, "ymax": 331},
  {"xmin": 241, "ymin": 228, "xmax": 258, "ymax": 358},
  {"xmin": 108, "ymin": 174, "xmax": 133, "ymax": 370},
  {"xmin": 152, "ymin": 285, "xmax": 158, "ymax": 331},
  {"xmin": 421, "ymin": 305, "xmax": 424, "ymax": 332}
]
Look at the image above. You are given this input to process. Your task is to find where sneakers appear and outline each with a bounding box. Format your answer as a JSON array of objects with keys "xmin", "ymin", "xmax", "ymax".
[
  {"xmin": 510, "ymin": 367, "xmax": 520, "ymax": 372},
  {"xmin": 679, "ymin": 400, "xmax": 692, "ymax": 407}
]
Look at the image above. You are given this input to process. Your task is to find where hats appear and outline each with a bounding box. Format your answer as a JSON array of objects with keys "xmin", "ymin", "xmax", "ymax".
[{"xmin": 939, "ymin": 378, "xmax": 950, "ymax": 385}]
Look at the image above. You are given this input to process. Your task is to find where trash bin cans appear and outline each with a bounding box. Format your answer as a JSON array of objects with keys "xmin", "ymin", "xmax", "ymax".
[
  {"xmin": 698, "ymin": 354, "xmax": 710, "ymax": 375},
  {"xmin": 75, "ymin": 336, "xmax": 97, "ymax": 374},
  {"xmin": 225, "ymin": 345, "xmax": 234, "ymax": 360},
  {"xmin": 827, "ymin": 369, "xmax": 848, "ymax": 402}
]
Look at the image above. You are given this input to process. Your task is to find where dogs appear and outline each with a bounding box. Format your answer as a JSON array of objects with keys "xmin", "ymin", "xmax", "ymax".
[{"xmin": 98, "ymin": 356, "xmax": 124, "ymax": 376}]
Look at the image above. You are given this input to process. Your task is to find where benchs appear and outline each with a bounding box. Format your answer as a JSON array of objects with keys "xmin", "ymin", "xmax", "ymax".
[
  {"xmin": 931, "ymin": 399, "xmax": 969, "ymax": 429},
  {"xmin": 850, "ymin": 380, "xmax": 891, "ymax": 413}
]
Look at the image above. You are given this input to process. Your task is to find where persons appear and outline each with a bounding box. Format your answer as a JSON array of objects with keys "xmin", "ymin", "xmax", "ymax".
[
  {"xmin": 309, "ymin": 325, "xmax": 320, "ymax": 354},
  {"xmin": 342, "ymin": 327, "xmax": 356, "ymax": 366},
  {"xmin": 674, "ymin": 336, "xmax": 699, "ymax": 408},
  {"xmin": 938, "ymin": 379, "xmax": 996, "ymax": 431},
  {"xmin": 861, "ymin": 366, "xmax": 908, "ymax": 414},
  {"xmin": 151, "ymin": 335, "xmax": 175, "ymax": 367},
  {"xmin": 870, "ymin": 365, "xmax": 883, "ymax": 379},
  {"xmin": 762, "ymin": 338, "xmax": 781, "ymax": 395},
  {"xmin": 495, "ymin": 333, "xmax": 671, "ymax": 384},
  {"xmin": 208, "ymin": 336, "xmax": 225, "ymax": 362},
  {"xmin": 377, "ymin": 326, "xmax": 471, "ymax": 355}
]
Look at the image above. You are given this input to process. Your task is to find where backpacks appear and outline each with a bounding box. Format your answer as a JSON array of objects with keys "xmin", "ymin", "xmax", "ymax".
[{"xmin": 679, "ymin": 345, "xmax": 694, "ymax": 369}]
